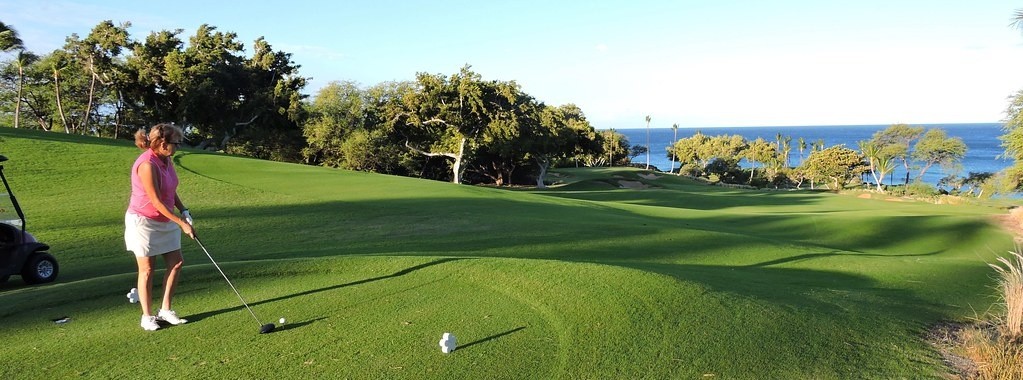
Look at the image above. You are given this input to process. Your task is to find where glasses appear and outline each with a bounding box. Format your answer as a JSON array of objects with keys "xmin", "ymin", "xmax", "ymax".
[{"xmin": 167, "ymin": 140, "xmax": 182, "ymax": 148}]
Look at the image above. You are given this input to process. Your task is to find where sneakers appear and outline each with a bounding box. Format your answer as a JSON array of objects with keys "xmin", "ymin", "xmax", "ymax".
[
  {"xmin": 158, "ymin": 309, "xmax": 182, "ymax": 325},
  {"xmin": 141, "ymin": 315, "xmax": 160, "ymax": 331}
]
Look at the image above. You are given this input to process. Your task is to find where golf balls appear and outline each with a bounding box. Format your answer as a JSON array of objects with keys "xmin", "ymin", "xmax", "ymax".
[{"xmin": 279, "ymin": 318, "xmax": 285, "ymax": 323}]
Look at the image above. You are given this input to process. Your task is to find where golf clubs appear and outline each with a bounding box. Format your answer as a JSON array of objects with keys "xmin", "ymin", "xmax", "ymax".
[{"xmin": 193, "ymin": 233, "xmax": 276, "ymax": 334}]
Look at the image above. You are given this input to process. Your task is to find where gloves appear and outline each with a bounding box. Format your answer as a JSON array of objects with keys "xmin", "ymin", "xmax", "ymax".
[{"xmin": 181, "ymin": 210, "xmax": 196, "ymax": 232}]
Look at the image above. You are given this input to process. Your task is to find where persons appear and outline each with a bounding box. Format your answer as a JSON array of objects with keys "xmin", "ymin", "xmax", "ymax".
[{"xmin": 124, "ymin": 124, "xmax": 196, "ymax": 331}]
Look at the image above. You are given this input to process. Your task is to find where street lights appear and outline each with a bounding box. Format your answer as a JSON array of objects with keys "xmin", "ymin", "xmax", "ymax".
[
  {"xmin": 609, "ymin": 128, "xmax": 617, "ymax": 167},
  {"xmin": 669, "ymin": 124, "xmax": 677, "ymax": 174},
  {"xmin": 645, "ymin": 115, "xmax": 652, "ymax": 170}
]
